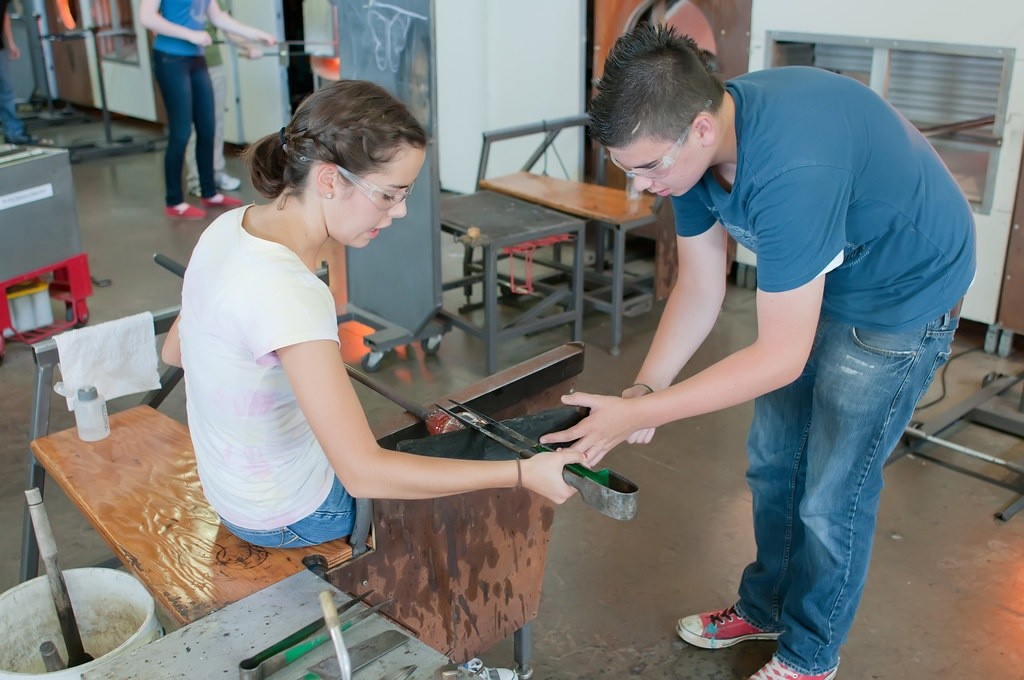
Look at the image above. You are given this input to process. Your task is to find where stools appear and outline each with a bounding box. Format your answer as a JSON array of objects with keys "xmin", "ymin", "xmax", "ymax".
[
  {"xmin": 30, "ymin": 401, "xmax": 358, "ymax": 631},
  {"xmin": 476, "ymin": 168, "xmax": 666, "ymax": 357},
  {"xmin": 441, "ymin": 189, "xmax": 587, "ymax": 375}
]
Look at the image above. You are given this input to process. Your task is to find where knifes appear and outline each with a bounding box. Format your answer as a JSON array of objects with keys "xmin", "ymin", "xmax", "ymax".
[{"xmin": 293, "ymin": 629, "xmax": 410, "ymax": 680}]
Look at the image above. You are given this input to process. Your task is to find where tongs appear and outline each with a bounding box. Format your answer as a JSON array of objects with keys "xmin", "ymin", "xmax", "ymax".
[
  {"xmin": 237, "ymin": 588, "xmax": 394, "ymax": 680},
  {"xmin": 435, "ymin": 399, "xmax": 640, "ymax": 521}
]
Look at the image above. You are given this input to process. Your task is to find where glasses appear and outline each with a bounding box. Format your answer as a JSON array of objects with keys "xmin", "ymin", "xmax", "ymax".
[
  {"xmin": 300, "ymin": 155, "xmax": 416, "ymax": 212},
  {"xmin": 608, "ymin": 99, "xmax": 713, "ymax": 182}
]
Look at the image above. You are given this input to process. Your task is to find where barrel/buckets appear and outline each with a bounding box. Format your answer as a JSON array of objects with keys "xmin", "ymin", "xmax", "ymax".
[{"xmin": 0, "ymin": 567, "xmax": 165, "ymax": 680}]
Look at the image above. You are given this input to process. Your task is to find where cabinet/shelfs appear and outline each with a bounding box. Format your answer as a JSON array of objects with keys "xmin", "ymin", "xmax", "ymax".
[{"xmin": 760, "ymin": 30, "xmax": 1023, "ymax": 328}]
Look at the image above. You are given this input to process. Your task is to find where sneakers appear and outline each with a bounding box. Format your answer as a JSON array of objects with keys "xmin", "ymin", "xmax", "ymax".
[
  {"xmin": 201, "ymin": 193, "xmax": 243, "ymax": 209},
  {"xmin": 749, "ymin": 655, "xmax": 840, "ymax": 680},
  {"xmin": 468, "ymin": 656, "xmax": 519, "ymax": 680},
  {"xmin": 215, "ymin": 173, "xmax": 242, "ymax": 191},
  {"xmin": 166, "ymin": 201, "xmax": 206, "ymax": 222},
  {"xmin": 675, "ymin": 604, "xmax": 785, "ymax": 650},
  {"xmin": 189, "ymin": 185, "xmax": 203, "ymax": 198}
]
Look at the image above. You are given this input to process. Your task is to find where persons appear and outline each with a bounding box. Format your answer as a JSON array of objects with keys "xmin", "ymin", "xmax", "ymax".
[
  {"xmin": 1, "ymin": 0, "xmax": 53, "ymax": 146},
  {"xmin": 161, "ymin": 78, "xmax": 589, "ymax": 679},
  {"xmin": 538, "ymin": 21, "xmax": 975, "ymax": 680},
  {"xmin": 184, "ymin": 0, "xmax": 263, "ymax": 199},
  {"xmin": 137, "ymin": 0, "xmax": 276, "ymax": 220}
]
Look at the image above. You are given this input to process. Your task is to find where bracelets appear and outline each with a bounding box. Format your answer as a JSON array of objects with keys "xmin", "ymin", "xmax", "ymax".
[
  {"xmin": 514, "ymin": 456, "xmax": 523, "ymax": 490},
  {"xmin": 633, "ymin": 383, "xmax": 655, "ymax": 393}
]
